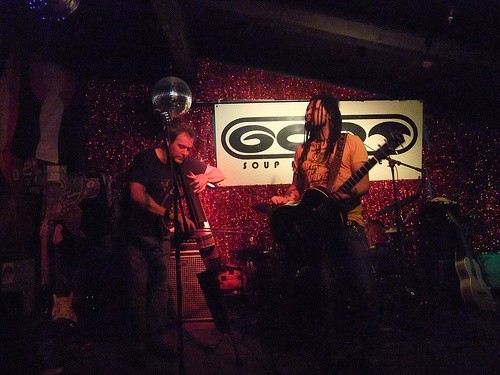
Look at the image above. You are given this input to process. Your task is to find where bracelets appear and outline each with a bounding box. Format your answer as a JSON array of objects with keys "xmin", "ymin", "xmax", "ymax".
[{"xmin": 164, "ymin": 209, "xmax": 172, "ymax": 220}]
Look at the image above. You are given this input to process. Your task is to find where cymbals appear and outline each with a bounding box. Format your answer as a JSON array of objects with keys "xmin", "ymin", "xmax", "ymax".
[{"xmin": 375, "ymin": 194, "xmax": 421, "ymax": 217}]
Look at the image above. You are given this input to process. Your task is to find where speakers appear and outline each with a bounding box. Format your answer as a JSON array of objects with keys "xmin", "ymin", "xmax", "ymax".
[{"xmin": 164, "ymin": 254, "xmax": 215, "ymax": 322}]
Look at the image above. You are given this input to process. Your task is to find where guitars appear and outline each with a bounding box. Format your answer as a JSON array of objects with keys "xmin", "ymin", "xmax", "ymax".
[
  {"xmin": 443, "ymin": 208, "xmax": 494, "ymax": 313},
  {"xmin": 268, "ymin": 130, "xmax": 406, "ymax": 250}
]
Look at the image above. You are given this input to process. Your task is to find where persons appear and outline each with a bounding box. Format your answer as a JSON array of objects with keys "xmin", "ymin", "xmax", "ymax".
[
  {"xmin": 273, "ymin": 93, "xmax": 369, "ymax": 336},
  {"xmin": 115, "ymin": 124, "xmax": 195, "ymax": 355}
]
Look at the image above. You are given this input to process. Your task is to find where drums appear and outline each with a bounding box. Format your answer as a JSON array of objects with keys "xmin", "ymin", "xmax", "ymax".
[{"xmin": 367, "ymin": 218, "xmax": 386, "ymax": 254}]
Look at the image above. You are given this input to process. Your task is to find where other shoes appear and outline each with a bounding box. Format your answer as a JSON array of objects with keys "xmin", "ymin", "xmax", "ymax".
[
  {"xmin": 325, "ymin": 347, "xmax": 347, "ymax": 363},
  {"xmin": 132, "ymin": 338, "xmax": 148, "ymax": 368},
  {"xmin": 364, "ymin": 351, "xmax": 381, "ymax": 370},
  {"xmin": 146, "ymin": 340, "xmax": 178, "ymax": 358}
]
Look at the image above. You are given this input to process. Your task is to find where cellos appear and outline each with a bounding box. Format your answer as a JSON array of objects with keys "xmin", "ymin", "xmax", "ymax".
[{"xmin": 154, "ymin": 97, "xmax": 244, "ymax": 368}]
